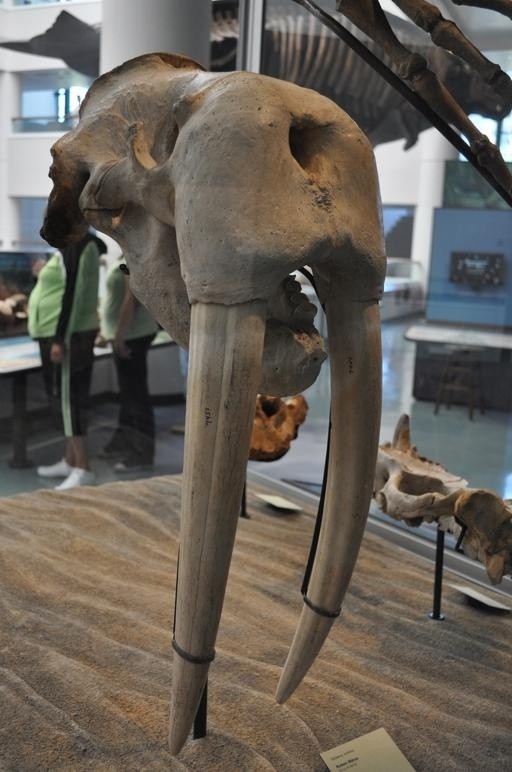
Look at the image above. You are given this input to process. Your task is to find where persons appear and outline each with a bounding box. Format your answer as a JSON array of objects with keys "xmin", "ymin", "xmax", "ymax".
[
  {"xmin": 27, "ymin": 230, "xmax": 109, "ymax": 492},
  {"xmin": 96, "ymin": 252, "xmax": 163, "ymax": 474}
]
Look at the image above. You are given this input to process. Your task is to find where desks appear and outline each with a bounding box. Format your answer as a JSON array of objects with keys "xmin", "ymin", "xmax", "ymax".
[
  {"xmin": 0, "ymin": 321, "xmax": 200, "ymax": 456},
  {"xmin": 404, "ymin": 325, "xmax": 508, "ymax": 414},
  {"xmin": 296, "ymin": 279, "xmax": 429, "ymax": 371}
]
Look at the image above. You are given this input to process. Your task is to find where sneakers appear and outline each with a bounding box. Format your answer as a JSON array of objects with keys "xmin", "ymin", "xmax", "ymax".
[
  {"xmin": 98, "ymin": 444, "xmax": 154, "ymax": 473},
  {"xmin": 54, "ymin": 466, "xmax": 98, "ymax": 491},
  {"xmin": 37, "ymin": 456, "xmax": 70, "ymax": 479}
]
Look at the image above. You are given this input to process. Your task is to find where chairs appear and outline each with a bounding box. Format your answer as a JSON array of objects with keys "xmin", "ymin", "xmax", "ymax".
[{"xmin": 433, "ymin": 346, "xmax": 486, "ymax": 421}]
